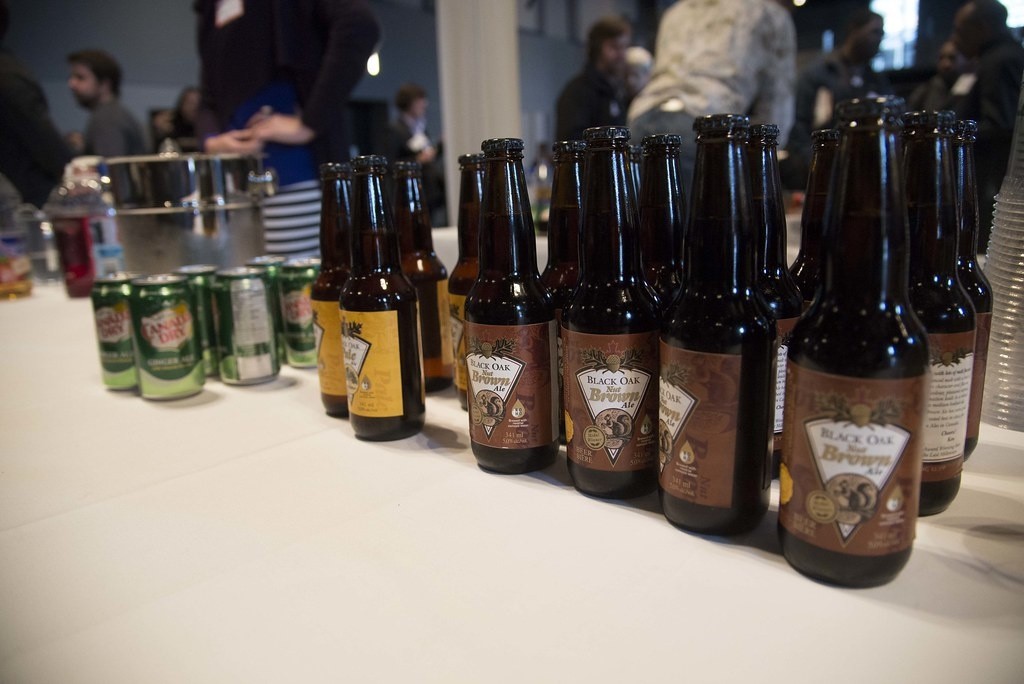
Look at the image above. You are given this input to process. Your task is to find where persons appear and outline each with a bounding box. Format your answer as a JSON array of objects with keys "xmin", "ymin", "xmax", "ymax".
[
  {"xmin": 389, "ymin": 82, "xmax": 443, "ymax": 172},
  {"xmin": 554, "ymin": 19, "xmax": 640, "ymax": 138},
  {"xmin": 912, "ymin": 0, "xmax": 1024, "ymax": 196},
  {"xmin": 67, "ymin": 47, "xmax": 145, "ymax": 157},
  {"xmin": 0, "ymin": 67, "xmax": 77, "ymax": 207},
  {"xmin": 188, "ymin": 0, "xmax": 381, "ymax": 261},
  {"xmin": 792, "ymin": 8, "xmax": 884, "ymax": 178},
  {"xmin": 152, "ymin": 86, "xmax": 206, "ymax": 153},
  {"xmin": 622, "ymin": 47, "xmax": 655, "ymax": 89},
  {"xmin": 624, "ymin": 0, "xmax": 795, "ymax": 212}
]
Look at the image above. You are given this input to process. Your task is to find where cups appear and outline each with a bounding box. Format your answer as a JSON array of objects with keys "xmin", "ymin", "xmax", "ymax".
[
  {"xmin": 0, "ymin": 206, "xmax": 96, "ymax": 301},
  {"xmin": 981, "ymin": 75, "xmax": 1024, "ymax": 433}
]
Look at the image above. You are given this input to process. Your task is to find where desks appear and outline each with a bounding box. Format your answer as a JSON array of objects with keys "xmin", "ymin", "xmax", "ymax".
[{"xmin": 0, "ymin": 227, "xmax": 1024, "ymax": 684}]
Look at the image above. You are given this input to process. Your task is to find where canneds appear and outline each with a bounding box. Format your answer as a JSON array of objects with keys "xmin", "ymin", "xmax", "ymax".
[{"xmin": 90, "ymin": 251, "xmax": 321, "ymax": 400}]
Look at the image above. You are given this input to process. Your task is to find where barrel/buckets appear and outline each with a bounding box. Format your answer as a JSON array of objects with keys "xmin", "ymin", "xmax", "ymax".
[{"xmin": 106, "ymin": 152, "xmax": 268, "ymax": 272}]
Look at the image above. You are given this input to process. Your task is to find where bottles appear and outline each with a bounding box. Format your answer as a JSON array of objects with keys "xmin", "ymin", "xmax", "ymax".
[
  {"xmin": 450, "ymin": 95, "xmax": 994, "ymax": 591},
  {"xmin": 309, "ymin": 155, "xmax": 448, "ymax": 442}
]
